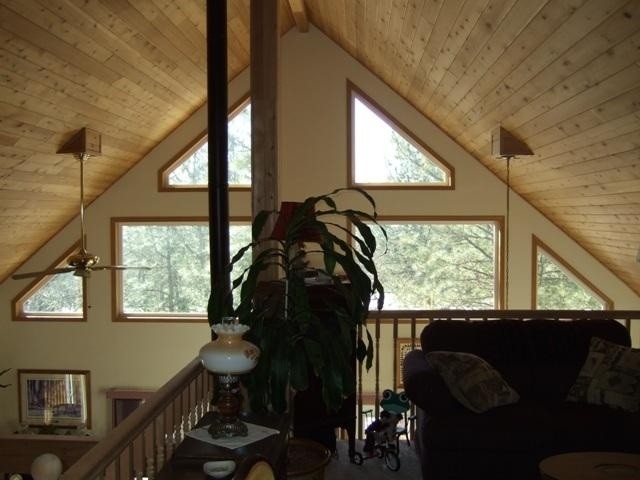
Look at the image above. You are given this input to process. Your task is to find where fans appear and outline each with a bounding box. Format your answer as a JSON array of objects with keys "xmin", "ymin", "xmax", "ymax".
[{"xmin": 13, "ymin": 130, "xmax": 154, "ymax": 281}]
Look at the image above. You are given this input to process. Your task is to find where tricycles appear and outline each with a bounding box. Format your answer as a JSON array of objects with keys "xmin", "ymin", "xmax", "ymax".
[{"xmin": 352, "ymin": 419, "xmax": 401, "ymax": 472}]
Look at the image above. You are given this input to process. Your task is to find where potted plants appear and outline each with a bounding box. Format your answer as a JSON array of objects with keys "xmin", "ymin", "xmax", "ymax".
[{"xmin": 218, "ymin": 189, "xmax": 385, "ymax": 480}]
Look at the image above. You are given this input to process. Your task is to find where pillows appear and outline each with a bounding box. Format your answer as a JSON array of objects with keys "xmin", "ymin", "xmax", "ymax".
[
  {"xmin": 566, "ymin": 337, "xmax": 640, "ymax": 413},
  {"xmin": 429, "ymin": 352, "xmax": 517, "ymax": 411}
]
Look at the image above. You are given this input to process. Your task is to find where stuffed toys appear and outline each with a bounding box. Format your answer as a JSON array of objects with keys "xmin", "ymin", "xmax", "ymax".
[{"xmin": 375, "ymin": 388, "xmax": 411, "ymax": 440}]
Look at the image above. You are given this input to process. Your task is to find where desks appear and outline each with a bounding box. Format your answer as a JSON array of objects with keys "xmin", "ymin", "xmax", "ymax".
[{"xmin": 535, "ymin": 450, "xmax": 640, "ymax": 480}]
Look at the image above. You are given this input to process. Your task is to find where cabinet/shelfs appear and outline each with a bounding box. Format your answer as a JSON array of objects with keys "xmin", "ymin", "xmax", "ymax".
[
  {"xmin": 0, "ymin": 435, "xmax": 101, "ymax": 479},
  {"xmin": 152, "ymin": 412, "xmax": 294, "ymax": 479}
]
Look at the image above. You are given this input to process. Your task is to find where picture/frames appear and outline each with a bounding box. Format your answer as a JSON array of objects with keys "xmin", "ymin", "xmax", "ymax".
[{"xmin": 15, "ymin": 368, "xmax": 93, "ymax": 430}]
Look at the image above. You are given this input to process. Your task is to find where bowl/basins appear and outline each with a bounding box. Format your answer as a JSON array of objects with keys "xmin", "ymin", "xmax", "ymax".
[{"xmin": 202, "ymin": 460, "xmax": 236, "ymax": 477}]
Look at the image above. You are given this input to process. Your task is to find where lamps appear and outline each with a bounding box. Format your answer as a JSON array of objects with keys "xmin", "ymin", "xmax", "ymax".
[
  {"xmin": 268, "ymin": 200, "xmax": 325, "ymax": 281},
  {"xmin": 199, "ymin": 318, "xmax": 262, "ymax": 439}
]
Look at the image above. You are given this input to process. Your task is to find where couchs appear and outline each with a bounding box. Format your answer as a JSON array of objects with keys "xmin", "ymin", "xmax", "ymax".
[{"xmin": 401, "ymin": 319, "xmax": 640, "ymax": 480}]
